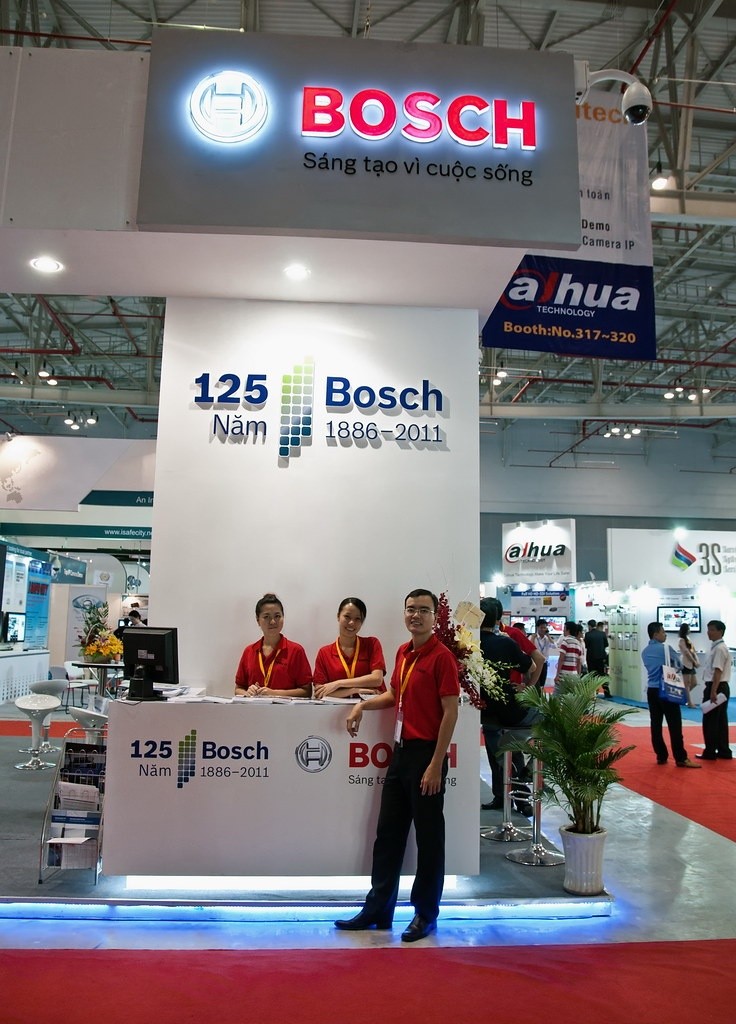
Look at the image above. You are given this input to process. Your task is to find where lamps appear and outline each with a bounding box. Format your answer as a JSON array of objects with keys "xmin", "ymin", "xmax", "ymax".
[
  {"xmin": 649, "ymin": 162, "xmax": 670, "ymax": 192},
  {"xmin": 38, "ymin": 359, "xmax": 59, "ymax": 388},
  {"xmin": 5, "ymin": 431, "xmax": 16, "ymax": 442},
  {"xmin": 86, "ymin": 408, "xmax": 100, "ymax": 427},
  {"xmin": 489, "ymin": 361, "xmax": 509, "ymax": 387},
  {"xmin": 603, "ymin": 422, "xmax": 642, "ymax": 442},
  {"xmin": 63, "ymin": 409, "xmax": 80, "ymax": 431}
]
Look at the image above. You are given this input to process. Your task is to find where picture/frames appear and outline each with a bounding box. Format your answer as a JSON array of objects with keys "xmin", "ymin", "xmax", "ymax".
[{"xmin": 608, "ymin": 613, "xmax": 639, "ymax": 651}]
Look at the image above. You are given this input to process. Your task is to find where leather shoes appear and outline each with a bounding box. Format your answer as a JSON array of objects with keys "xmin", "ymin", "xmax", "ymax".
[
  {"xmin": 481, "ymin": 799, "xmax": 514, "ymax": 810},
  {"xmin": 335, "ymin": 908, "xmax": 393, "ymax": 929},
  {"xmin": 517, "ymin": 803, "xmax": 534, "ymax": 817},
  {"xmin": 401, "ymin": 915, "xmax": 438, "ymax": 942}
]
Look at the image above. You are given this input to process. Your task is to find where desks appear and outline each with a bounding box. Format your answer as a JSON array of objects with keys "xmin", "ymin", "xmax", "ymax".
[{"xmin": 73, "ymin": 659, "xmax": 126, "ymax": 697}]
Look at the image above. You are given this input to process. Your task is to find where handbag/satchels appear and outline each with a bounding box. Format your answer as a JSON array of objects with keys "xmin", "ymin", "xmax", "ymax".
[{"xmin": 659, "ymin": 643, "xmax": 687, "ymax": 705}]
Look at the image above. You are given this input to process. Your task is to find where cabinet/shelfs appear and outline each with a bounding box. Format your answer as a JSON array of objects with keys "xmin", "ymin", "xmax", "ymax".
[{"xmin": 35, "ymin": 725, "xmax": 108, "ymax": 889}]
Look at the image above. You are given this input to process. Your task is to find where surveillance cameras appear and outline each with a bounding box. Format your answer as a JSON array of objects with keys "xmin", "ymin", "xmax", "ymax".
[
  {"xmin": 53, "ymin": 560, "xmax": 61, "ymax": 571},
  {"xmin": 622, "ymin": 82, "xmax": 654, "ymax": 125}
]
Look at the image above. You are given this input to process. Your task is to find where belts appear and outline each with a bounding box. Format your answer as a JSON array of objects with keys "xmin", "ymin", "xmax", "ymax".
[{"xmin": 395, "ymin": 738, "xmax": 439, "ymax": 748}]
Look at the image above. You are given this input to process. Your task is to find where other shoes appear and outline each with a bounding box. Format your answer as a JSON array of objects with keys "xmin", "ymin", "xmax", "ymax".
[
  {"xmin": 657, "ymin": 759, "xmax": 667, "ymax": 764},
  {"xmin": 676, "ymin": 758, "xmax": 702, "ymax": 768},
  {"xmin": 696, "ymin": 753, "xmax": 704, "ymax": 759}
]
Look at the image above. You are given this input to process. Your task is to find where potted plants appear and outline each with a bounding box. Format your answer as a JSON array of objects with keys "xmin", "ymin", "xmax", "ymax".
[{"xmin": 502, "ymin": 672, "xmax": 641, "ymax": 898}]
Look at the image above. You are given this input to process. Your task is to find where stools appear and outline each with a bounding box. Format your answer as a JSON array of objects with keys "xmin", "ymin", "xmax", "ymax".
[{"xmin": 481, "ymin": 726, "xmax": 566, "ymax": 867}]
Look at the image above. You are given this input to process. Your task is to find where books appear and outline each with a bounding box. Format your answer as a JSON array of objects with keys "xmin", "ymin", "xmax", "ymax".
[{"xmin": 122, "ymin": 681, "xmax": 382, "ymax": 705}]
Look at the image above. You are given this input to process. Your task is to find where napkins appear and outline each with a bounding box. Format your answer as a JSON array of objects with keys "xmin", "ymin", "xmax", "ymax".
[{"xmin": 15, "ymin": 681, "xmax": 107, "ymax": 772}]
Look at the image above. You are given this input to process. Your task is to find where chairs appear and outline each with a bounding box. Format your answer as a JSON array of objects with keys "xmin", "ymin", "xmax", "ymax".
[{"xmin": 47, "ymin": 658, "xmax": 97, "ymax": 715}]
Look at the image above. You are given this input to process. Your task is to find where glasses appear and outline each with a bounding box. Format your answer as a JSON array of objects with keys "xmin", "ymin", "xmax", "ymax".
[{"xmin": 405, "ymin": 608, "xmax": 436, "ymax": 615}]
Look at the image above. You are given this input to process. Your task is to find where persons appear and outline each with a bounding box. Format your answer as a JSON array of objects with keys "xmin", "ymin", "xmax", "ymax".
[
  {"xmin": 676, "ymin": 623, "xmax": 701, "ymax": 709},
  {"xmin": 695, "ymin": 620, "xmax": 732, "ymax": 760},
  {"xmin": 312, "ymin": 597, "xmax": 388, "ymax": 699},
  {"xmin": 641, "ymin": 622, "xmax": 702, "ymax": 768},
  {"xmin": 232, "ymin": 593, "xmax": 313, "ymax": 698},
  {"xmin": 334, "ymin": 589, "xmax": 460, "ymax": 942},
  {"xmin": 477, "ymin": 596, "xmax": 615, "ymax": 818},
  {"xmin": 114, "ymin": 610, "xmax": 146, "ymax": 640}
]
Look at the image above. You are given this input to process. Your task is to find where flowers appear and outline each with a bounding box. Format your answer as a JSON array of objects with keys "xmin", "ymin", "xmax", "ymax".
[{"xmin": 77, "ymin": 627, "xmax": 125, "ymax": 655}]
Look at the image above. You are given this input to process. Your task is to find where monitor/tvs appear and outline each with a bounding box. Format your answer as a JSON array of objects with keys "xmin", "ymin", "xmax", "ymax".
[
  {"xmin": 539, "ymin": 615, "xmax": 567, "ymax": 635},
  {"xmin": 510, "ymin": 615, "xmax": 537, "ymax": 634},
  {"xmin": 657, "ymin": 606, "xmax": 701, "ymax": 633},
  {"xmin": 123, "ymin": 627, "xmax": 179, "ymax": 700},
  {"xmin": 3, "ymin": 612, "xmax": 26, "ymax": 642}
]
